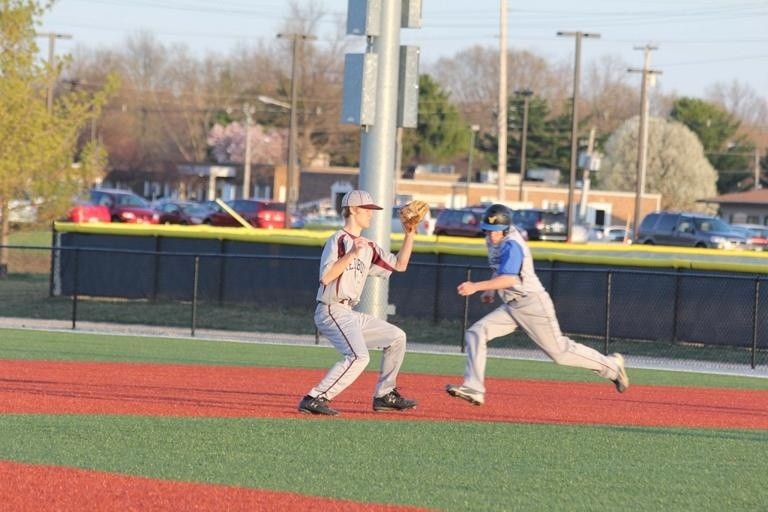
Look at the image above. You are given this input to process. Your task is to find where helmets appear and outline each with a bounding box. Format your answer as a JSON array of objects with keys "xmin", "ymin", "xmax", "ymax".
[{"xmin": 480, "ymin": 204, "xmax": 512, "ymax": 237}]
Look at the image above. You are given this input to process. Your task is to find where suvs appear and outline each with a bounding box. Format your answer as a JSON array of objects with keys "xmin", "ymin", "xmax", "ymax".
[
  {"xmin": 513, "ymin": 208, "xmax": 570, "ymax": 242},
  {"xmin": 636, "ymin": 212, "xmax": 747, "ymax": 250}
]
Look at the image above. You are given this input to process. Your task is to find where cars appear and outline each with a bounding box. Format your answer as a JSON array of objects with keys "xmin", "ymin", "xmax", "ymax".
[
  {"xmin": 560, "ymin": 208, "xmax": 635, "ymax": 243},
  {"xmin": 87, "ymin": 188, "xmax": 160, "ymax": 226},
  {"xmin": 0, "ymin": 187, "xmax": 37, "ymax": 229},
  {"xmin": 154, "ymin": 199, "xmax": 210, "ymax": 227},
  {"xmin": 208, "ymin": 199, "xmax": 293, "ymax": 231},
  {"xmin": 36, "ymin": 199, "xmax": 111, "ymax": 224},
  {"xmin": 433, "ymin": 208, "xmax": 529, "ymax": 243},
  {"xmin": 731, "ymin": 224, "xmax": 768, "ymax": 252},
  {"xmin": 391, "ymin": 205, "xmax": 438, "ymax": 236},
  {"xmin": 291, "ymin": 198, "xmax": 348, "ymax": 231}
]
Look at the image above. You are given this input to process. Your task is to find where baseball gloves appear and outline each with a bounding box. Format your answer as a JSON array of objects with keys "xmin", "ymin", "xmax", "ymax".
[{"xmin": 399, "ymin": 199, "xmax": 428, "ymax": 232}]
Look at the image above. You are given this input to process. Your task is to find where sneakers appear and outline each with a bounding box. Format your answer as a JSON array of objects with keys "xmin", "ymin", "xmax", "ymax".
[
  {"xmin": 298, "ymin": 394, "xmax": 340, "ymax": 416},
  {"xmin": 609, "ymin": 352, "xmax": 630, "ymax": 393},
  {"xmin": 373, "ymin": 388, "xmax": 419, "ymax": 412},
  {"xmin": 446, "ymin": 383, "xmax": 485, "ymax": 406}
]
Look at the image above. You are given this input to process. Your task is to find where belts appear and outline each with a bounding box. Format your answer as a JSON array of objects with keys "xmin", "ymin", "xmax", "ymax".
[{"xmin": 509, "ymin": 294, "xmax": 527, "ymax": 303}]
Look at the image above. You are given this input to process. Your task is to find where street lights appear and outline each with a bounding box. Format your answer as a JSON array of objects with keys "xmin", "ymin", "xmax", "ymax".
[
  {"xmin": 33, "ymin": 32, "xmax": 73, "ymax": 118},
  {"xmin": 514, "ymin": 89, "xmax": 535, "ymax": 203},
  {"xmin": 465, "ymin": 124, "xmax": 482, "ymax": 209},
  {"xmin": 626, "ymin": 68, "xmax": 665, "ymax": 228},
  {"xmin": 275, "ymin": 32, "xmax": 319, "ymax": 230},
  {"xmin": 557, "ymin": 30, "xmax": 602, "ymax": 224}
]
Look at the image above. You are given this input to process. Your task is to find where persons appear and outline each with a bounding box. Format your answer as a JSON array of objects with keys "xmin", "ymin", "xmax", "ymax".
[
  {"xmin": 445, "ymin": 204, "xmax": 630, "ymax": 405},
  {"xmin": 297, "ymin": 190, "xmax": 429, "ymax": 416}
]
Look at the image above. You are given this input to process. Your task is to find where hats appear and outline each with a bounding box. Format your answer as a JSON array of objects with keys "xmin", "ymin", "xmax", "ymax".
[{"xmin": 341, "ymin": 190, "xmax": 384, "ymax": 210}]
